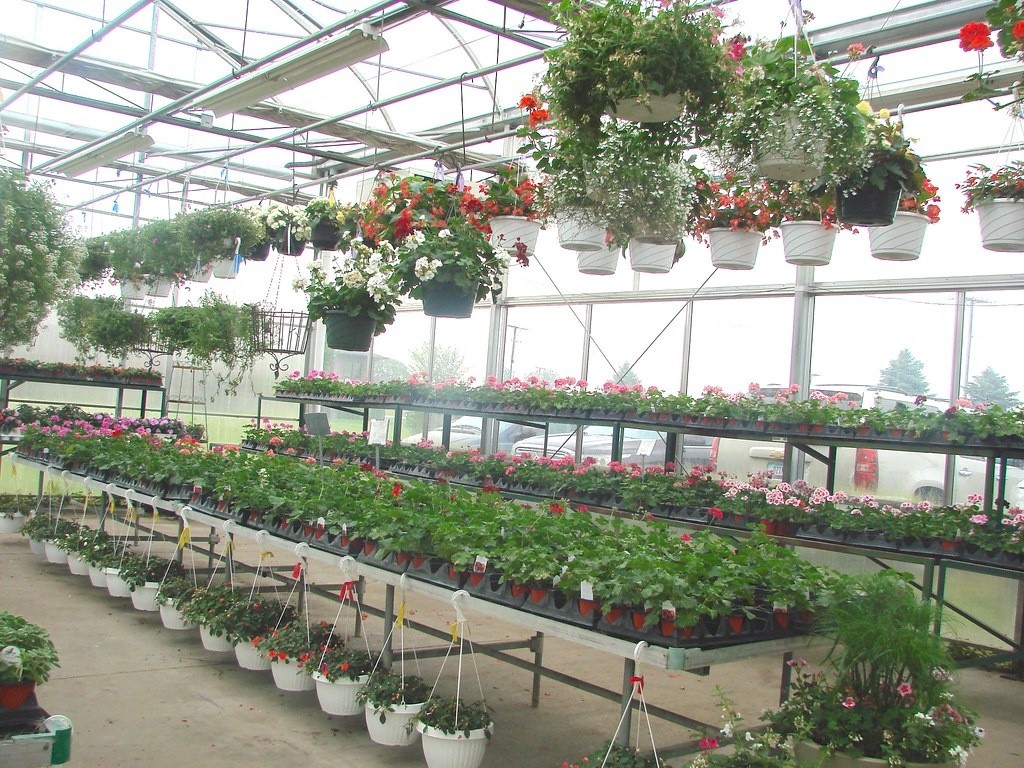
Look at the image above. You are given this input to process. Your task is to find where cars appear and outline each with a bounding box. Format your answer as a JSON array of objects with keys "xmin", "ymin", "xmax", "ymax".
[
  {"xmin": 514, "ymin": 422, "xmax": 713, "ymax": 474},
  {"xmin": 401, "ymin": 411, "xmax": 577, "ymax": 461}
]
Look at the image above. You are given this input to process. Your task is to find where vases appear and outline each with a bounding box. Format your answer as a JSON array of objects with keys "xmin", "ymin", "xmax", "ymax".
[{"xmin": 0, "ymin": 94, "xmax": 1023, "ymax": 766}]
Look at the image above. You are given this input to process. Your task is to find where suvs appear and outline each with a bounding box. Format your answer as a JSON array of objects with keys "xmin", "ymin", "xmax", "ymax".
[{"xmin": 711, "ymin": 382, "xmax": 1024, "ymax": 511}]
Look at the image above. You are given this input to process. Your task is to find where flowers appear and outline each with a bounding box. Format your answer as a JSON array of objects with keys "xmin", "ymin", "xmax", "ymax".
[
  {"xmin": 401, "ymin": 694, "xmax": 496, "ymax": 744},
  {"xmin": 1, "ymin": 0, "xmax": 1024, "ymax": 682},
  {"xmin": 780, "ymin": 580, "xmax": 984, "ymax": 768},
  {"xmin": 354, "ymin": 666, "xmax": 442, "ymax": 725}
]
